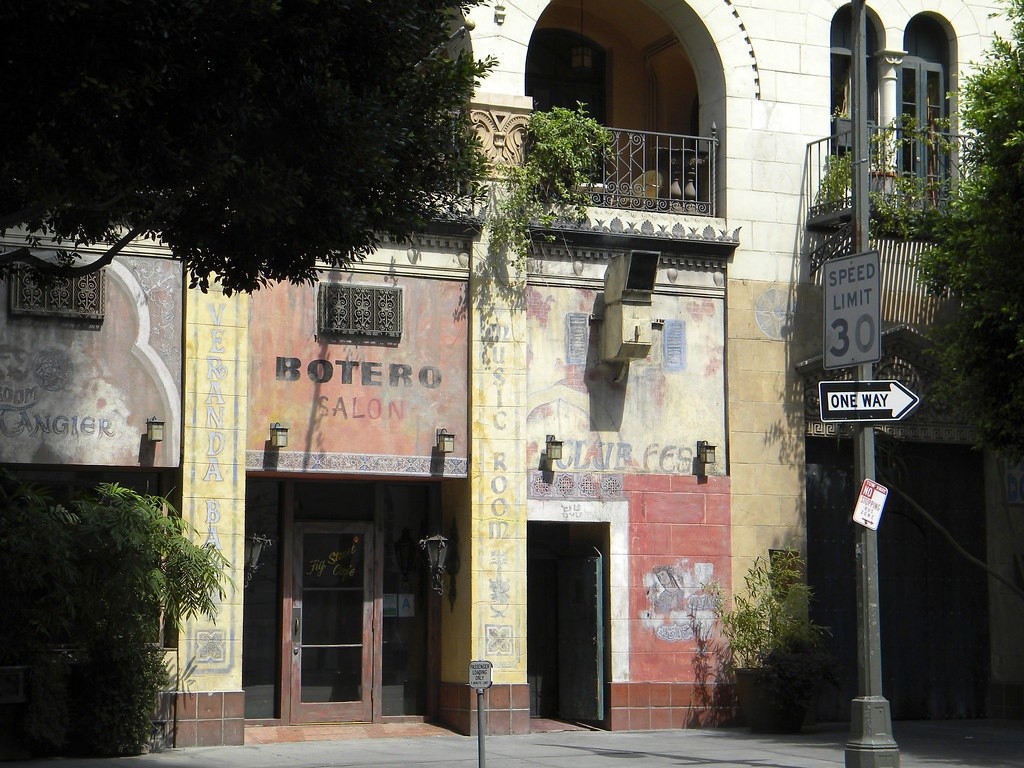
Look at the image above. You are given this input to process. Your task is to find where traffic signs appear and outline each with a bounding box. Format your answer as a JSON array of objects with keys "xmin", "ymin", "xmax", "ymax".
[{"xmin": 817, "ymin": 377, "xmax": 921, "ymax": 422}]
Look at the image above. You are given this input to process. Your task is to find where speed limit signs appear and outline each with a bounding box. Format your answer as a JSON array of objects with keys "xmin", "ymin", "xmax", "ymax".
[{"xmin": 821, "ymin": 249, "xmax": 884, "ymax": 371}]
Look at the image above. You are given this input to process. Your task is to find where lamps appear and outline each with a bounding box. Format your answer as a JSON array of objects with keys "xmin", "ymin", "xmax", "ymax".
[
  {"xmin": 436, "ymin": 428, "xmax": 455, "ymax": 453},
  {"xmin": 545, "ymin": 435, "xmax": 564, "ymax": 460},
  {"xmin": 697, "ymin": 440, "xmax": 717, "ymax": 464},
  {"xmin": 418, "ymin": 529, "xmax": 459, "ymax": 601},
  {"xmin": 244, "ymin": 533, "xmax": 273, "ymax": 588},
  {"xmin": 146, "ymin": 416, "xmax": 166, "ymax": 443},
  {"xmin": 269, "ymin": 422, "xmax": 290, "ymax": 448}
]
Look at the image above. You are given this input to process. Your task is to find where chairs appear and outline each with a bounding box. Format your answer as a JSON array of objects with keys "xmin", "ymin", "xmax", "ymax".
[{"xmin": 633, "ymin": 171, "xmax": 662, "ymax": 199}]
